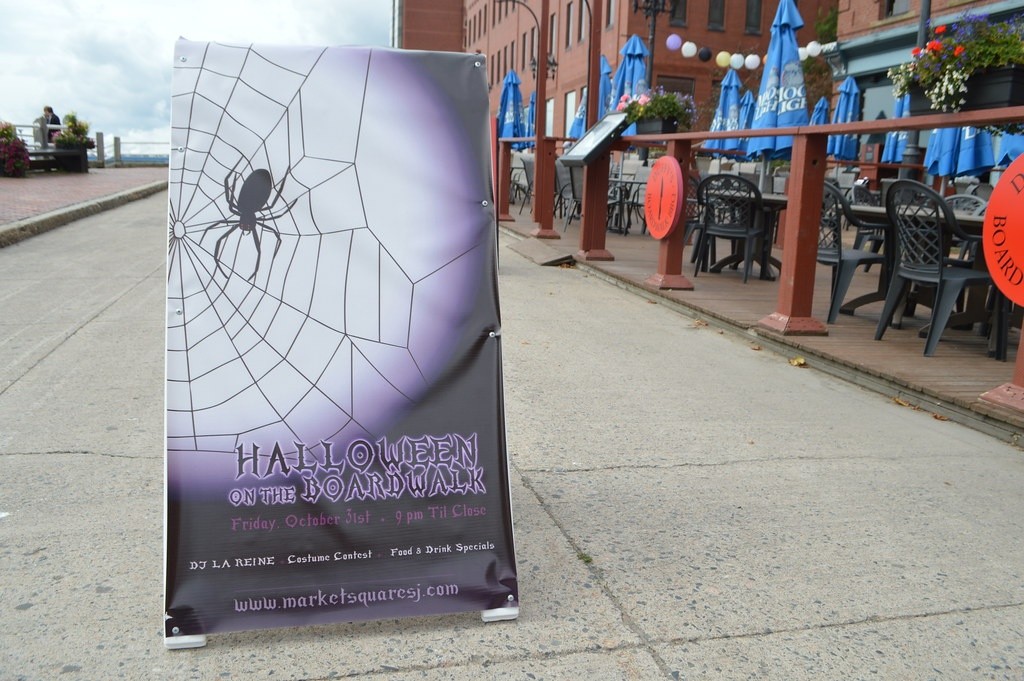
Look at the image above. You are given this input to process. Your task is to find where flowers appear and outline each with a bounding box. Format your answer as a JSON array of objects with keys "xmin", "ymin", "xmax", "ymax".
[
  {"xmin": 886, "ymin": 7, "xmax": 1024, "ymax": 115},
  {"xmin": 616, "ymin": 81, "xmax": 705, "ymax": 132},
  {"xmin": 51, "ymin": 113, "xmax": 98, "ymax": 149},
  {"xmin": 0, "ymin": 119, "xmax": 30, "ymax": 177}
]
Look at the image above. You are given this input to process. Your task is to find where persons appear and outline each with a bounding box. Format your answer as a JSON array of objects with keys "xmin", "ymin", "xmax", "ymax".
[{"xmin": 42, "ymin": 107, "xmax": 60, "ymax": 142}]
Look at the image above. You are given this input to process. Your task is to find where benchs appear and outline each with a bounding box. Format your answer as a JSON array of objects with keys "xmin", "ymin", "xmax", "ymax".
[{"xmin": 27, "ymin": 149, "xmax": 87, "ymax": 172}]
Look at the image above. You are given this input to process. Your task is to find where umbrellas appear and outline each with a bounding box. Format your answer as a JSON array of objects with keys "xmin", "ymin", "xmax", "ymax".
[
  {"xmin": 702, "ymin": 0, "xmax": 1024, "ymax": 200},
  {"xmin": 499, "ymin": 35, "xmax": 650, "ymax": 149}
]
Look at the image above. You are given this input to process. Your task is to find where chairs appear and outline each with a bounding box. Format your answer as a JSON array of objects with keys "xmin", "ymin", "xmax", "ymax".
[{"xmin": 510, "ymin": 157, "xmax": 1024, "ymax": 363}]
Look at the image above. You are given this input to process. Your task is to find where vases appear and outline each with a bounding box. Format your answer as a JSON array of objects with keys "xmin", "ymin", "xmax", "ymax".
[
  {"xmin": 971, "ymin": 64, "xmax": 1024, "ymax": 109},
  {"xmin": 637, "ymin": 115, "xmax": 678, "ymax": 135},
  {"xmin": 909, "ymin": 81, "xmax": 971, "ymax": 115}
]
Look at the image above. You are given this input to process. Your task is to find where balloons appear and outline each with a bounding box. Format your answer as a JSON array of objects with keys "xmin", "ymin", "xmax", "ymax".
[{"xmin": 666, "ymin": 34, "xmax": 820, "ymax": 69}]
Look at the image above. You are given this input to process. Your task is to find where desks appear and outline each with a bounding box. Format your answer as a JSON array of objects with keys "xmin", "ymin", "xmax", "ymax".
[
  {"xmin": 610, "ymin": 178, "xmax": 647, "ymax": 233},
  {"xmin": 704, "ymin": 188, "xmax": 788, "ymax": 276},
  {"xmin": 832, "ymin": 205, "xmax": 1017, "ymax": 337}
]
